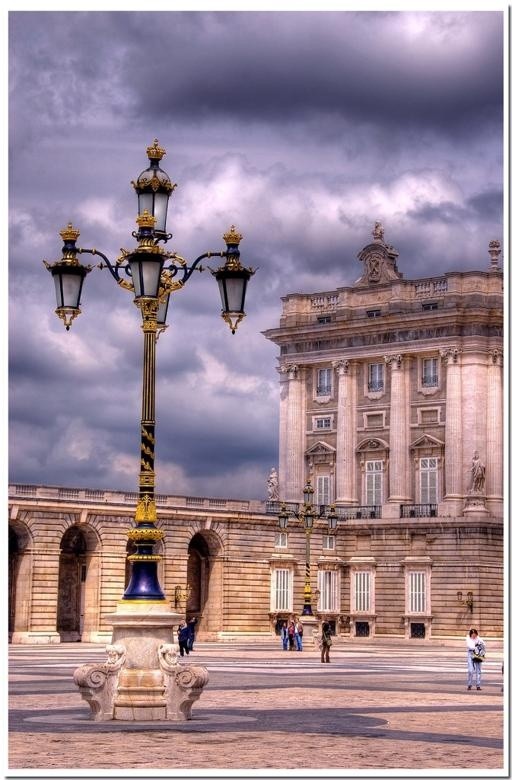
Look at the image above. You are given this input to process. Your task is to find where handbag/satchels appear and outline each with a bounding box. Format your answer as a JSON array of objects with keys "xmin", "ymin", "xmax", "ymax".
[
  {"xmin": 472, "ymin": 651, "xmax": 485, "ymax": 663},
  {"xmin": 324, "ymin": 639, "xmax": 332, "ymax": 647}
]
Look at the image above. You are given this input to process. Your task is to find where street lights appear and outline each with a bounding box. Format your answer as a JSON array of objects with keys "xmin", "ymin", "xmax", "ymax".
[
  {"xmin": 40, "ymin": 139, "xmax": 260, "ymax": 729},
  {"xmin": 275, "ymin": 477, "xmax": 342, "ymax": 648}
]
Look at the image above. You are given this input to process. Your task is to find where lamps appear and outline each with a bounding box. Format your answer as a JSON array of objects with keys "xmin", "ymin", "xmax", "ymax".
[
  {"xmin": 311, "ymin": 589, "xmax": 320, "ymax": 607},
  {"xmin": 172, "ymin": 583, "xmax": 193, "ymax": 605},
  {"xmin": 456, "ymin": 588, "xmax": 475, "ymax": 616}
]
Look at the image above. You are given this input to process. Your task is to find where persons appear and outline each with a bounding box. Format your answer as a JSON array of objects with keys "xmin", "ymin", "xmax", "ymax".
[
  {"xmin": 279, "ymin": 617, "xmax": 304, "ymax": 652},
  {"xmin": 466, "ymin": 629, "xmax": 486, "ymax": 690},
  {"xmin": 320, "ymin": 622, "xmax": 333, "ymax": 663},
  {"xmin": 177, "ymin": 619, "xmax": 191, "ymax": 658},
  {"xmin": 187, "ymin": 616, "xmax": 199, "ymax": 651}
]
